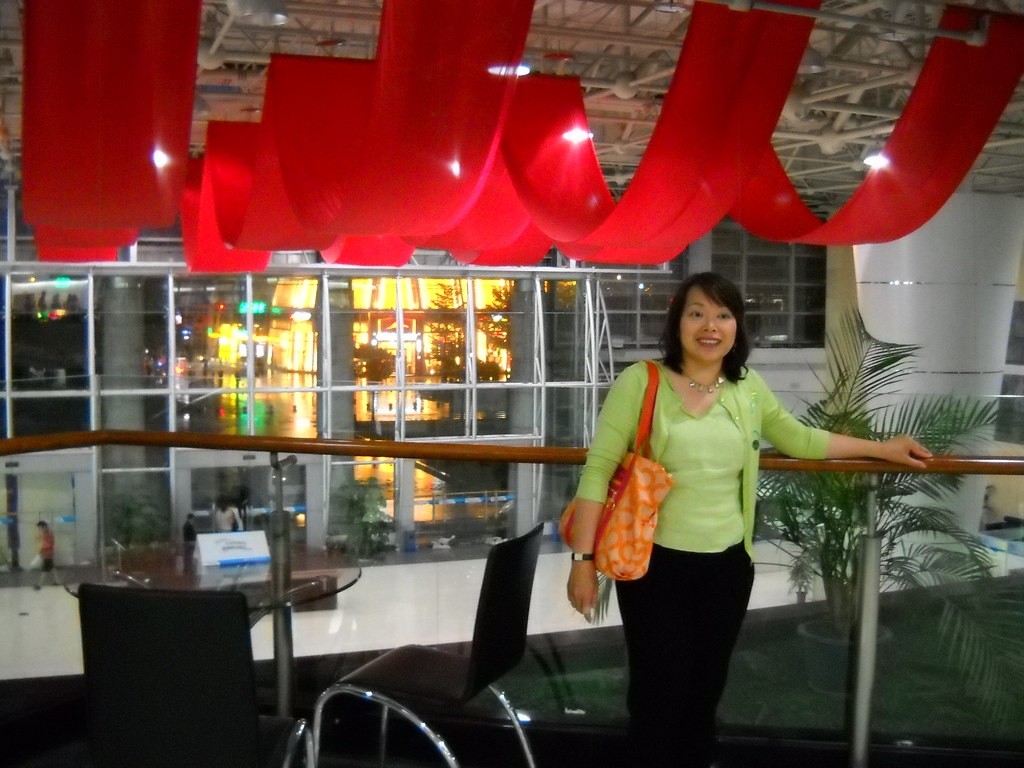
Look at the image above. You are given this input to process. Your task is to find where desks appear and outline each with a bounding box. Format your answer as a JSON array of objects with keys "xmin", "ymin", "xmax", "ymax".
[{"xmin": 51, "ymin": 540, "xmax": 363, "ymax": 718}]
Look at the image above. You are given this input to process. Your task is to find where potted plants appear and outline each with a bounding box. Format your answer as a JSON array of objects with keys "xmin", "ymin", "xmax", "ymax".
[{"xmin": 756, "ymin": 302, "xmax": 1016, "ymax": 731}]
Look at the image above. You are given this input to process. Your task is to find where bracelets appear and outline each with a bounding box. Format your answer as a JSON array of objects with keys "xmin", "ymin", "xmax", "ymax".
[{"xmin": 571, "ymin": 552, "xmax": 593, "ymax": 561}]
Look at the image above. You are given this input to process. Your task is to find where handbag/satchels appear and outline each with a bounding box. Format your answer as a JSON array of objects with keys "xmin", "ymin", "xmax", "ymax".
[{"xmin": 557, "ymin": 358, "xmax": 674, "ymax": 579}]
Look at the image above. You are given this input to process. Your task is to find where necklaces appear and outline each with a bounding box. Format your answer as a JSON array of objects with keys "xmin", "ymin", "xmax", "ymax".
[{"xmin": 684, "ymin": 372, "xmax": 724, "ymax": 393}]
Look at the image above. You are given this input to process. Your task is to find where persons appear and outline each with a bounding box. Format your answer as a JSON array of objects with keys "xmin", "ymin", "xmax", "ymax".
[
  {"xmin": 567, "ymin": 273, "xmax": 933, "ymax": 727},
  {"xmin": 32, "ymin": 520, "xmax": 61, "ymax": 590},
  {"xmin": 360, "ymin": 476, "xmax": 386, "ymax": 558},
  {"xmin": 183, "ymin": 513, "xmax": 197, "ymax": 543},
  {"xmin": 215, "ymin": 495, "xmax": 236, "ymax": 532}
]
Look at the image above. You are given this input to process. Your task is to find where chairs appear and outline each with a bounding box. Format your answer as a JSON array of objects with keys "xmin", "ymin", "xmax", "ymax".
[
  {"xmin": 307, "ymin": 521, "xmax": 545, "ymax": 768},
  {"xmin": 76, "ymin": 584, "xmax": 314, "ymax": 768}
]
